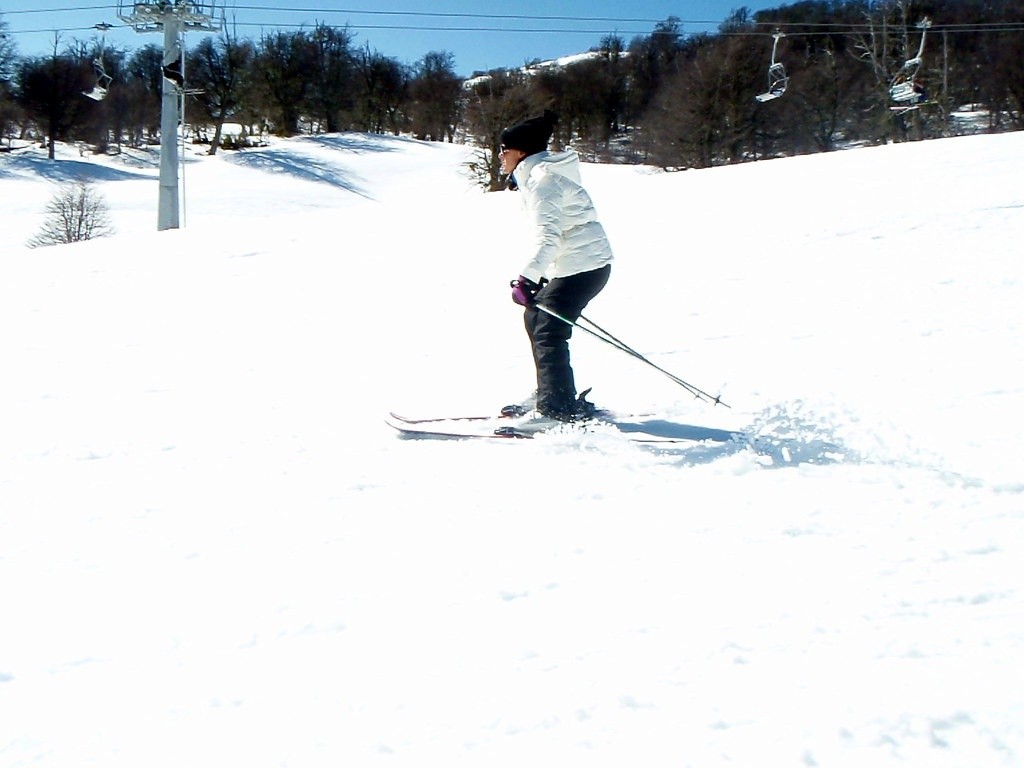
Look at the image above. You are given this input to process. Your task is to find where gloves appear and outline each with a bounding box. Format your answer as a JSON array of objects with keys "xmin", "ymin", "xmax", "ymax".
[{"xmin": 512, "ymin": 275, "xmax": 536, "ymax": 307}]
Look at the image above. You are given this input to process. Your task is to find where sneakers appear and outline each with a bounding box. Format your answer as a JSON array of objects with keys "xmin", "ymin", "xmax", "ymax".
[
  {"xmin": 507, "ymin": 410, "xmax": 557, "ymax": 432},
  {"xmin": 519, "ymin": 393, "xmax": 538, "ymax": 411}
]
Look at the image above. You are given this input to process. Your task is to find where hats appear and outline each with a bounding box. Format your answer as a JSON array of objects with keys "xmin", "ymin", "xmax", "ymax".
[{"xmin": 500, "ymin": 115, "xmax": 555, "ymax": 153}]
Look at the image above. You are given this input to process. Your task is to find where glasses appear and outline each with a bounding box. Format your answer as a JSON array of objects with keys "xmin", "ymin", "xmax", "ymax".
[{"xmin": 499, "ymin": 145, "xmax": 509, "ymax": 153}]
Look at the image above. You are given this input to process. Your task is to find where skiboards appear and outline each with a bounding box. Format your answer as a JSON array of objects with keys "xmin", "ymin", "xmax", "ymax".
[{"xmin": 384, "ymin": 408, "xmax": 696, "ymax": 444}]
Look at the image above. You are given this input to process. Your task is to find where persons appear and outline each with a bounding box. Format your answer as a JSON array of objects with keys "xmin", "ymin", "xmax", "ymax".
[{"xmin": 499, "ymin": 109, "xmax": 615, "ymax": 430}]
